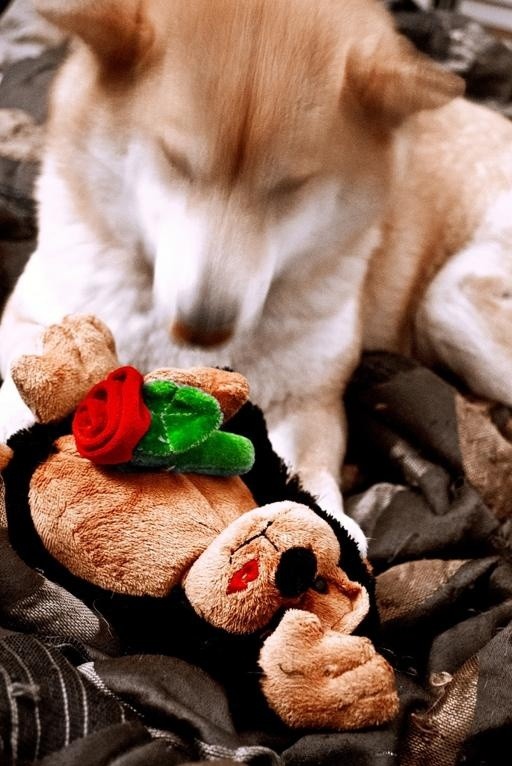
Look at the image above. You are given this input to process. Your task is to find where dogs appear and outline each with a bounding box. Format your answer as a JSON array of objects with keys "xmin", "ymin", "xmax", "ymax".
[{"xmin": 0, "ymin": 0, "xmax": 511, "ymax": 573}]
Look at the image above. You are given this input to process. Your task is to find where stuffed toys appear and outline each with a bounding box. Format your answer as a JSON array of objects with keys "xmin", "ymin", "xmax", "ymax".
[{"xmin": 0, "ymin": 314, "xmax": 404, "ymax": 734}]
